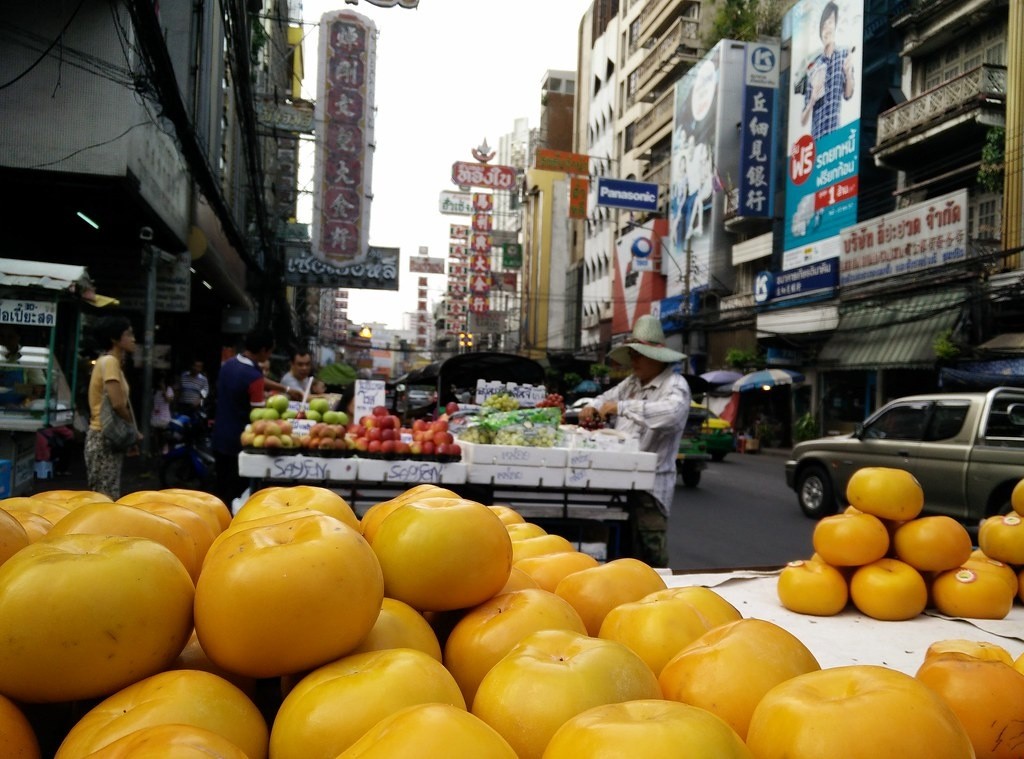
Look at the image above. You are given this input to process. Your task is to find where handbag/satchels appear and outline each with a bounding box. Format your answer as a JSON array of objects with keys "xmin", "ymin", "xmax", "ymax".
[{"xmin": 100, "ymin": 355, "xmax": 139, "ymax": 451}]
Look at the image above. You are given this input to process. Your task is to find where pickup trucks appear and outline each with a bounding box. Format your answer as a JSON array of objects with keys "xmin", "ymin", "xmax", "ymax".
[{"xmin": 785, "ymin": 386, "xmax": 1024, "ymax": 530}]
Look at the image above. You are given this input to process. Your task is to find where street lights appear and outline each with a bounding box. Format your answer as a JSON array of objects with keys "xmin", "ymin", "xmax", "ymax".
[{"xmin": 627, "ymin": 220, "xmax": 690, "ymax": 374}]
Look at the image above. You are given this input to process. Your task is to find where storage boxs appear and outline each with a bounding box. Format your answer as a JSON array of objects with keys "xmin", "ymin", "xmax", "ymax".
[{"xmin": 232, "ymin": 401, "xmax": 657, "ymax": 562}]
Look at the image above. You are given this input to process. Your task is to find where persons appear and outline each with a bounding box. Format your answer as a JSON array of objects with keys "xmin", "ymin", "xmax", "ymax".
[
  {"xmin": 578, "ymin": 314, "xmax": 691, "ymax": 570},
  {"xmin": 147, "ymin": 329, "xmax": 326, "ymax": 511},
  {"xmin": 84, "ymin": 312, "xmax": 137, "ymax": 501},
  {"xmin": 799, "ymin": 1, "xmax": 855, "ymax": 140}
]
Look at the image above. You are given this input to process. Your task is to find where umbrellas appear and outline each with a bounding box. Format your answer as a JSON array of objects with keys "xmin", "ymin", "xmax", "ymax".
[
  {"xmin": 691, "ymin": 370, "xmax": 743, "ymax": 410},
  {"xmin": 731, "ymin": 368, "xmax": 806, "ymax": 447}
]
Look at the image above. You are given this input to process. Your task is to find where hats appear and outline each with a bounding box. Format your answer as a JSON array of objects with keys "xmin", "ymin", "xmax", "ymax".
[{"xmin": 604, "ymin": 314, "xmax": 690, "ymax": 367}]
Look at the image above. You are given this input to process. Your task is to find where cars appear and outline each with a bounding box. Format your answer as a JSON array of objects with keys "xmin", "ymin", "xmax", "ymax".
[{"xmin": 685, "ymin": 399, "xmax": 737, "ymax": 464}]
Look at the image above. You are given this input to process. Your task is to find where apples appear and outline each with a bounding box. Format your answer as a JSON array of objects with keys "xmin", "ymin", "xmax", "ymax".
[{"xmin": 241, "ymin": 395, "xmax": 461, "ymax": 457}]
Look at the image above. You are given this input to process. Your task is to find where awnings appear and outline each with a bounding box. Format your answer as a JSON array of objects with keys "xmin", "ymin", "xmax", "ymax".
[
  {"xmin": 938, "ymin": 358, "xmax": 1024, "ymax": 382},
  {"xmin": 816, "ymin": 285, "xmax": 973, "ymax": 372},
  {"xmin": 969, "ymin": 332, "xmax": 1023, "ymax": 354}
]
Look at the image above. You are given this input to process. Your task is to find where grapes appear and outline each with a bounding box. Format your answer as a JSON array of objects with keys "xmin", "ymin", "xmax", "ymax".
[{"xmin": 459, "ymin": 392, "xmax": 605, "ymax": 447}]
